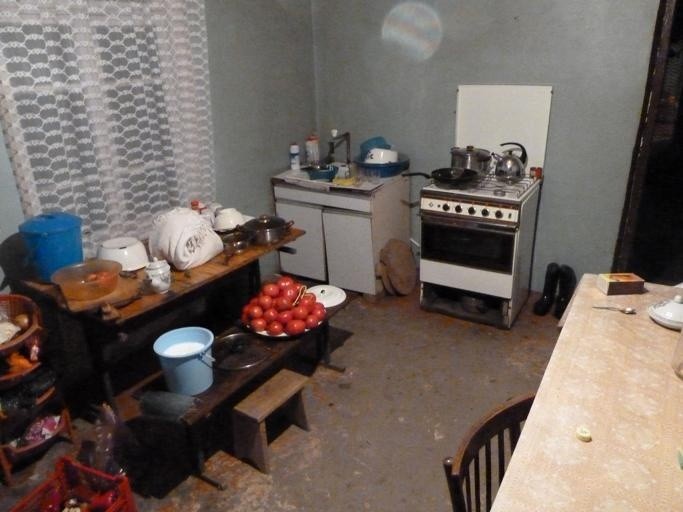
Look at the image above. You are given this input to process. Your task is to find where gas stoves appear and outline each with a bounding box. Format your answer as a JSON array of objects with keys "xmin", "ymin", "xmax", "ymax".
[{"xmin": 418, "ymin": 175, "xmax": 541, "ymax": 224}]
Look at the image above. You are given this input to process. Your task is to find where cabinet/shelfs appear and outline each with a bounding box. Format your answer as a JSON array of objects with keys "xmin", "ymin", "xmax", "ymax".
[
  {"xmin": 0, "ymin": 340, "xmax": 77, "ymax": 490},
  {"xmin": 272, "ymin": 184, "xmax": 413, "ymax": 298}
]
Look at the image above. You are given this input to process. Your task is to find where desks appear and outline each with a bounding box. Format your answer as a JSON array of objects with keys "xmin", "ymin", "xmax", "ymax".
[
  {"xmin": 18, "ymin": 213, "xmax": 307, "ymax": 417},
  {"xmin": 488, "ymin": 272, "xmax": 683, "ymax": 511}
]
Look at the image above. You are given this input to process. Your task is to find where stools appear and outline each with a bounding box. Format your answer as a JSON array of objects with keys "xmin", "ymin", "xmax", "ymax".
[{"xmin": 229, "ymin": 362, "xmax": 311, "ymax": 476}]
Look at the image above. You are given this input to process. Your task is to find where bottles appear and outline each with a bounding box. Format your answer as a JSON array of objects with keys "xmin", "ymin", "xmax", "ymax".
[
  {"xmin": 143, "ymin": 254, "xmax": 172, "ymax": 296},
  {"xmin": 289, "ymin": 142, "xmax": 301, "ymax": 173},
  {"xmin": 305, "ymin": 134, "xmax": 320, "ymax": 166}
]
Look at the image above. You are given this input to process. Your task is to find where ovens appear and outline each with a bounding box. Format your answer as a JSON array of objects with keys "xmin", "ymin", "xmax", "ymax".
[{"xmin": 416, "ymin": 210, "xmax": 520, "ymax": 301}]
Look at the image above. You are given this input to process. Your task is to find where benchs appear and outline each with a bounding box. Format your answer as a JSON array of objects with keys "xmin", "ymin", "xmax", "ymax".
[{"xmin": 131, "ymin": 280, "xmax": 359, "ymax": 492}]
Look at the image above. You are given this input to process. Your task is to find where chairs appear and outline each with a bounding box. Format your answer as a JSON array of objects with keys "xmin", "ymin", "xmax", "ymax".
[{"xmin": 441, "ymin": 390, "xmax": 534, "ymax": 511}]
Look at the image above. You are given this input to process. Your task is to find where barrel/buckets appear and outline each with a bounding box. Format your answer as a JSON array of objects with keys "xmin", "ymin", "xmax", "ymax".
[
  {"xmin": 18, "ymin": 211, "xmax": 85, "ymax": 283},
  {"xmin": 153, "ymin": 327, "xmax": 217, "ymax": 397}
]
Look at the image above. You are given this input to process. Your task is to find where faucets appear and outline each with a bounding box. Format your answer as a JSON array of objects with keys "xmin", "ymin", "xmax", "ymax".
[{"xmin": 327, "ymin": 132, "xmax": 351, "ymax": 164}]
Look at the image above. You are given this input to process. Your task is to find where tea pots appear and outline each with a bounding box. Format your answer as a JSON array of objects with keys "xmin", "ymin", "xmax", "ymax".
[{"xmin": 491, "ymin": 141, "xmax": 529, "ymax": 183}]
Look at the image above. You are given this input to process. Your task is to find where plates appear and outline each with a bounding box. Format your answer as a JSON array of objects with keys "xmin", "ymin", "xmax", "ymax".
[{"xmin": 242, "ymin": 319, "xmax": 326, "ymax": 340}]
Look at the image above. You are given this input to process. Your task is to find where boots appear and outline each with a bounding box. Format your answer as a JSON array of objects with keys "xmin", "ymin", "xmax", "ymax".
[
  {"xmin": 532, "ymin": 263, "xmax": 561, "ymax": 317},
  {"xmin": 552, "ymin": 265, "xmax": 577, "ymax": 320}
]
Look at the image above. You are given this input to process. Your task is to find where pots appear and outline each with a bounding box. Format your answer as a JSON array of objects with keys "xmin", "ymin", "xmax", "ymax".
[
  {"xmin": 236, "ymin": 214, "xmax": 296, "ymax": 246},
  {"xmin": 457, "ymin": 292, "xmax": 490, "ymax": 316},
  {"xmin": 400, "ymin": 167, "xmax": 479, "ymax": 191},
  {"xmin": 448, "ymin": 145, "xmax": 492, "ymax": 177}
]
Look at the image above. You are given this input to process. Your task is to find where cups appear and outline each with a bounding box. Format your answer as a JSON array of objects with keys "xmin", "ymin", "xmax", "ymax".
[
  {"xmin": 530, "ymin": 167, "xmax": 542, "ymax": 178},
  {"xmin": 349, "ymin": 166, "xmax": 383, "ymax": 188}
]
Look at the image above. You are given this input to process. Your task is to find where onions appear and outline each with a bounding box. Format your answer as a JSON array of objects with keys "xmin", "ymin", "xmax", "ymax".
[
  {"xmin": 86, "ymin": 271, "xmax": 115, "ymax": 286},
  {"xmin": 14, "ymin": 314, "xmax": 30, "ymax": 329}
]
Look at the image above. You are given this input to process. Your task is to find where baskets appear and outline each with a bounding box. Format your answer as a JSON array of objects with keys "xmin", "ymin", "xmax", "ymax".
[{"xmin": 7, "ymin": 454, "xmax": 138, "ymax": 512}]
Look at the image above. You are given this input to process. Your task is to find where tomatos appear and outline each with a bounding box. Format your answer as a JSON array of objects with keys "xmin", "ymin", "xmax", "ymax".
[{"xmin": 240, "ymin": 277, "xmax": 326, "ymax": 336}]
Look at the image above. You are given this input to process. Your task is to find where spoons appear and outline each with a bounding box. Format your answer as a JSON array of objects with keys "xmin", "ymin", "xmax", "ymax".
[{"xmin": 593, "ymin": 305, "xmax": 637, "ymax": 316}]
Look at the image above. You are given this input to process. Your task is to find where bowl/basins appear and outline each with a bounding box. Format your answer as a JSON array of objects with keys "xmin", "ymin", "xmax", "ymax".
[
  {"xmin": 48, "ymin": 258, "xmax": 120, "ymax": 305},
  {"xmin": 350, "ymin": 135, "xmax": 410, "ymax": 178},
  {"xmin": 95, "ymin": 237, "xmax": 149, "ymax": 273},
  {"xmin": 189, "ymin": 199, "xmax": 246, "ymax": 230},
  {"xmin": 223, "ymin": 233, "xmax": 251, "ymax": 255},
  {"xmin": 305, "ymin": 163, "xmax": 339, "ymax": 181}
]
